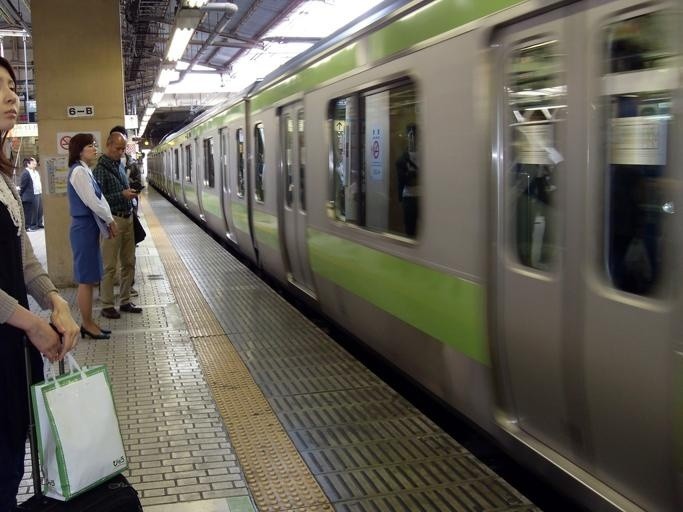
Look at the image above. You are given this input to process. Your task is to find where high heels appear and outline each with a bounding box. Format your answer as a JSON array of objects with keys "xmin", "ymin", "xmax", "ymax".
[{"xmin": 80, "ymin": 328, "xmax": 112, "ymax": 339}]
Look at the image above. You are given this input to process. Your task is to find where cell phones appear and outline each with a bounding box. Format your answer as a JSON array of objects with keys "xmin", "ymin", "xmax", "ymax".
[{"xmin": 135, "ymin": 186, "xmax": 145, "ymax": 193}]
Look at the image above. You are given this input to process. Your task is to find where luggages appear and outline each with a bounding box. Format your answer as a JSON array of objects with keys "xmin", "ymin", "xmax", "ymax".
[{"xmin": 19, "ymin": 322, "xmax": 142, "ymax": 511}]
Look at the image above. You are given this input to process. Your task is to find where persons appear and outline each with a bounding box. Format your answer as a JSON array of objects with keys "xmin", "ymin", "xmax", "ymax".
[
  {"xmin": 30, "ymin": 157, "xmax": 43, "ymax": 228},
  {"xmin": 94, "ymin": 132, "xmax": 141, "ymax": 318},
  {"xmin": 67, "ymin": 133, "xmax": 118, "ymax": 339},
  {"xmin": 0, "ymin": 52, "xmax": 80, "ymax": 510},
  {"xmin": 396, "ymin": 122, "xmax": 417, "ymax": 237},
  {"xmin": 19, "ymin": 158, "xmax": 34, "ymax": 227},
  {"xmin": 110, "ymin": 127, "xmax": 145, "ymax": 296}
]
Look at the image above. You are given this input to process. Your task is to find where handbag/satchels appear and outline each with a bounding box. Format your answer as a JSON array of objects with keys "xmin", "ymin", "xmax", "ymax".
[
  {"xmin": 32, "ymin": 350, "xmax": 127, "ymax": 500},
  {"xmin": 133, "ymin": 211, "xmax": 145, "ymax": 244}
]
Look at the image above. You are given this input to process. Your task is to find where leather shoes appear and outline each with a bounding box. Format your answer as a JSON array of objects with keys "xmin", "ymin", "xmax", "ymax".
[
  {"xmin": 121, "ymin": 304, "xmax": 142, "ymax": 313},
  {"xmin": 102, "ymin": 307, "xmax": 120, "ymax": 318}
]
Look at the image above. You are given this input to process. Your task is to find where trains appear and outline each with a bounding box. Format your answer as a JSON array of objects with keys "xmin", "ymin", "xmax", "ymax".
[{"xmin": 146, "ymin": 0, "xmax": 683, "ymax": 512}]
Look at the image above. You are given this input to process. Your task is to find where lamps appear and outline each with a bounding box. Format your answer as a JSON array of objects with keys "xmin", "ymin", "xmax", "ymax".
[{"xmin": 136, "ymin": 0, "xmax": 207, "ymax": 115}]
